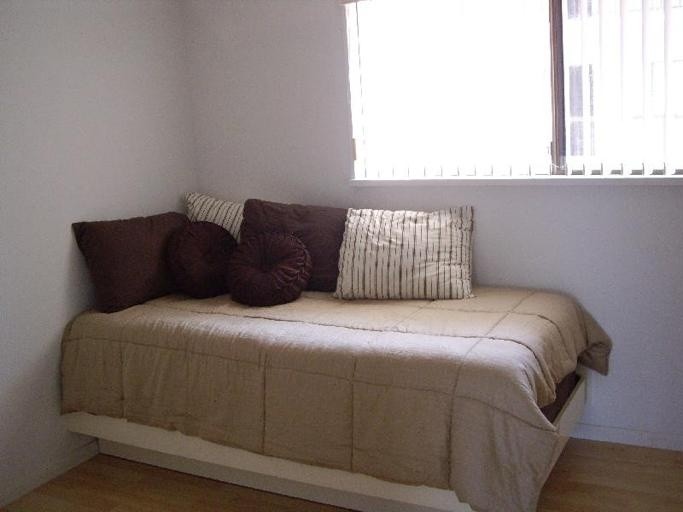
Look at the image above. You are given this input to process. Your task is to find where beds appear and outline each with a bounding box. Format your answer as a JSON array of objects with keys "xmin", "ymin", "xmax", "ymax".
[{"xmin": 60, "ymin": 286, "xmax": 589, "ymax": 512}]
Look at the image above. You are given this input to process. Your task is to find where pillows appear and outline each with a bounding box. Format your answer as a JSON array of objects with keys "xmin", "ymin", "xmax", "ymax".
[
  {"xmin": 237, "ymin": 195, "xmax": 348, "ymax": 294},
  {"xmin": 74, "ymin": 210, "xmax": 192, "ymax": 312},
  {"xmin": 181, "ymin": 193, "xmax": 246, "ymax": 237},
  {"xmin": 161, "ymin": 220, "xmax": 238, "ymax": 292},
  {"xmin": 227, "ymin": 231, "xmax": 314, "ymax": 307},
  {"xmin": 331, "ymin": 205, "xmax": 472, "ymax": 302}
]
